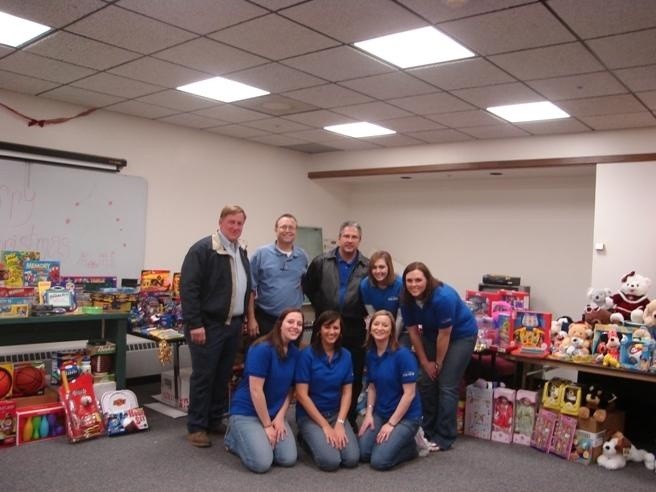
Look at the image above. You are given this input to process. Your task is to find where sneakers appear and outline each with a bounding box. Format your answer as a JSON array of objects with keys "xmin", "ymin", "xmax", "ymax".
[
  {"xmin": 208, "ymin": 422, "xmax": 227, "ymax": 435},
  {"xmin": 414, "ymin": 425, "xmax": 431, "ymax": 457},
  {"xmin": 185, "ymin": 430, "xmax": 211, "ymax": 447},
  {"xmin": 428, "ymin": 441, "xmax": 440, "ymax": 451}
]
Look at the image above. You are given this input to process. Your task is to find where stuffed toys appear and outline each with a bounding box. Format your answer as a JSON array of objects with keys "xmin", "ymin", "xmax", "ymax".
[
  {"xmin": 579, "ymin": 382, "xmax": 607, "ymax": 422},
  {"xmin": 551, "ymin": 316, "xmax": 573, "ymax": 352},
  {"xmin": 620, "ymin": 328, "xmax": 656, "ymax": 375},
  {"xmin": 560, "ymin": 322, "xmax": 592, "ymax": 356},
  {"xmin": 597, "ymin": 430, "xmax": 656, "ymax": 470},
  {"xmin": 583, "ymin": 276, "xmax": 611, "ymax": 322},
  {"xmin": 514, "ymin": 312, "xmax": 545, "ymax": 347},
  {"xmin": 606, "ymin": 272, "xmax": 656, "ymax": 325},
  {"xmin": 602, "ymin": 330, "xmax": 621, "ymax": 367}
]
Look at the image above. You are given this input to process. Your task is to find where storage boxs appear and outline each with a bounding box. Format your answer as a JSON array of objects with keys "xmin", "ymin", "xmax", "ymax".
[
  {"xmin": 572, "ymin": 432, "xmax": 625, "ymax": 463},
  {"xmin": 159, "ymin": 367, "xmax": 193, "ymax": 410}
]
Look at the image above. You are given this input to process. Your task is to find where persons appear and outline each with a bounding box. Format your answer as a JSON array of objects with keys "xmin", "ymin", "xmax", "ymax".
[
  {"xmin": 246, "ymin": 214, "xmax": 310, "ymax": 354},
  {"xmin": 400, "ymin": 263, "xmax": 478, "ymax": 451},
  {"xmin": 358, "ymin": 310, "xmax": 431, "ymax": 470},
  {"xmin": 303, "ymin": 222, "xmax": 370, "ymax": 434},
  {"xmin": 493, "ymin": 396, "xmax": 512, "ymax": 433},
  {"xmin": 295, "ymin": 310, "xmax": 360, "ymax": 472},
  {"xmin": 360, "ymin": 252, "xmax": 411, "ymax": 350},
  {"xmin": 180, "ymin": 206, "xmax": 250, "ymax": 447},
  {"xmin": 515, "ymin": 397, "xmax": 534, "ymax": 435},
  {"xmin": 224, "ymin": 309, "xmax": 307, "ymax": 474}
]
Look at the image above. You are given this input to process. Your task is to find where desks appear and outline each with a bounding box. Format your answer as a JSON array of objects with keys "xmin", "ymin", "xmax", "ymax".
[
  {"xmin": 128, "ymin": 325, "xmax": 188, "ymax": 409},
  {"xmin": 504, "ymin": 349, "xmax": 656, "ymax": 395},
  {"xmin": 470, "ymin": 342, "xmax": 523, "ymax": 386},
  {"xmin": 0, "ymin": 309, "xmax": 131, "ymax": 390}
]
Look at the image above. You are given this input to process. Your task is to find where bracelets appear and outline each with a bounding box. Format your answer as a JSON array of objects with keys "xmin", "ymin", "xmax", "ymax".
[
  {"xmin": 263, "ymin": 424, "xmax": 273, "ymax": 428},
  {"xmin": 336, "ymin": 419, "xmax": 344, "ymax": 425},
  {"xmin": 366, "ymin": 405, "xmax": 375, "ymax": 409},
  {"xmin": 386, "ymin": 421, "xmax": 397, "ymax": 429}
]
